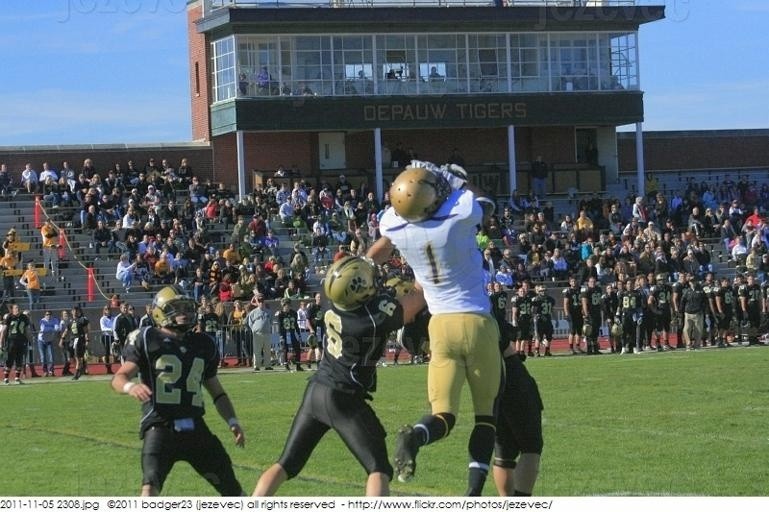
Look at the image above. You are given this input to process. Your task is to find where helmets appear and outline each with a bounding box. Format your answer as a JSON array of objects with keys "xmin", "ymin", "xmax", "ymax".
[
  {"xmin": 325, "ymin": 256, "xmax": 380, "ymax": 311},
  {"xmin": 151, "ymin": 284, "xmax": 199, "ymax": 334},
  {"xmin": 379, "ymin": 274, "xmax": 417, "ymax": 325},
  {"xmin": 389, "ymin": 167, "xmax": 452, "ymax": 224},
  {"xmin": 537, "ymin": 285, "xmax": 548, "ymax": 298}
]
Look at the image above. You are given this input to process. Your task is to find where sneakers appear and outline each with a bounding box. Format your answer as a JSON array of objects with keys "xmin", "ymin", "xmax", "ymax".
[{"xmin": 394, "ymin": 424, "xmax": 417, "ymax": 484}]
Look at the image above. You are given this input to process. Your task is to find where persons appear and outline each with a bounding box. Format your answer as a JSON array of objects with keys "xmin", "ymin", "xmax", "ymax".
[
  {"xmin": 382, "ymin": 191, "xmax": 390, "ymax": 209},
  {"xmin": 109, "ymin": 285, "xmax": 248, "ymax": 496},
  {"xmin": 1, "ymin": 156, "xmax": 76, "ymax": 208},
  {"xmin": 238, "ymin": 65, "xmax": 445, "ymax": 93},
  {"xmin": 670, "ymin": 176, "xmax": 769, "ymax": 232},
  {"xmin": 0, "ymin": 227, "xmax": 21, "ymax": 311},
  {"xmin": 196, "ymin": 294, "xmax": 325, "ymax": 371},
  {"xmin": 485, "ymin": 282, "xmax": 556, "ymax": 356},
  {"xmin": 252, "ymin": 236, "xmax": 425, "ymax": 496},
  {"xmin": 563, "ymin": 273, "xmax": 768, "ymax": 355},
  {"xmin": 727, "ymin": 232, "xmax": 769, "ymax": 276},
  {"xmin": 41, "ymin": 218, "xmax": 62, "ymax": 285},
  {"xmin": 481, "ymin": 155, "xmax": 713, "ymax": 286},
  {"xmin": 1, "ymin": 302, "xmax": 156, "ymax": 385},
  {"xmin": 556, "ymin": 66, "xmax": 626, "ymax": 90},
  {"xmin": 19, "ymin": 259, "xmax": 40, "ymax": 311},
  {"xmin": 379, "ymin": 158, "xmax": 502, "ymax": 497},
  {"xmin": 398, "ymin": 277, "xmax": 544, "ymax": 496}
]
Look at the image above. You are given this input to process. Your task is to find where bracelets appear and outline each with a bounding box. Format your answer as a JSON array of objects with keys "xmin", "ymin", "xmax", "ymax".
[{"xmin": 213, "ymin": 393, "xmax": 226, "ymax": 404}]
